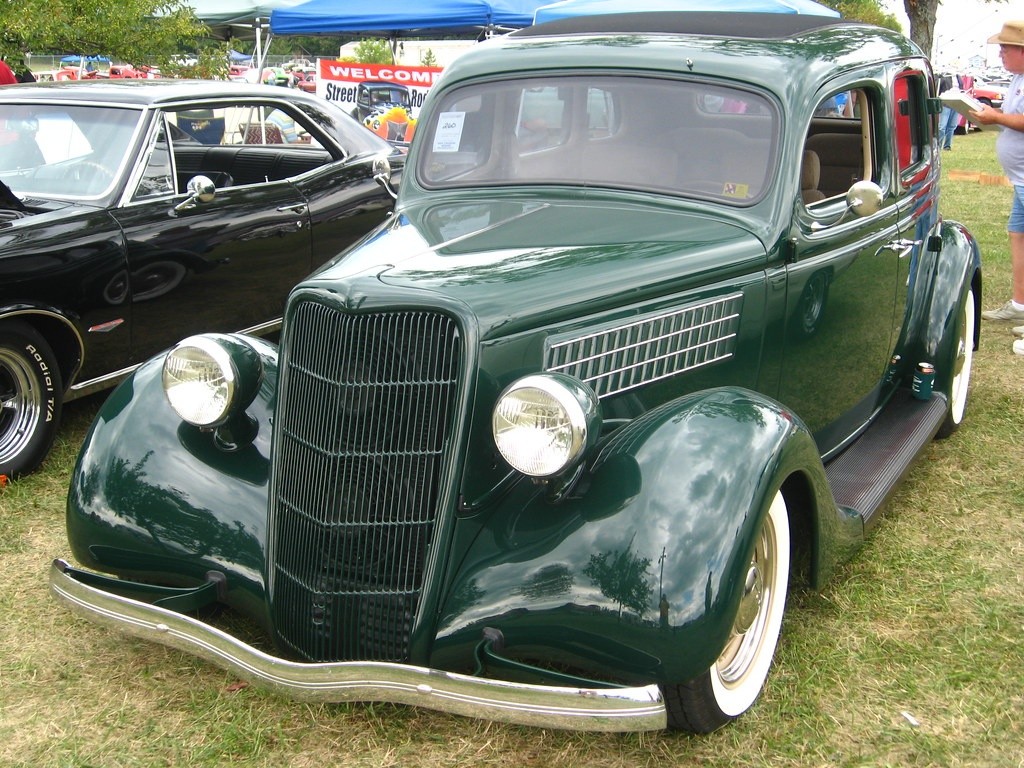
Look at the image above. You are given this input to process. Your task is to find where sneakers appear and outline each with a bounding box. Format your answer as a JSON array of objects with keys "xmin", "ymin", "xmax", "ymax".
[
  {"xmin": 1012, "ymin": 326, "xmax": 1024, "ymax": 334},
  {"xmin": 982, "ymin": 301, "xmax": 1024, "ymax": 320}
]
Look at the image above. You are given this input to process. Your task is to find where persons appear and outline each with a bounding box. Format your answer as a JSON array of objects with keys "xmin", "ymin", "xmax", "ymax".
[
  {"xmin": 937, "ymin": 66, "xmax": 964, "ymax": 150},
  {"xmin": 968, "ymin": 20, "xmax": 1024, "ymax": 337},
  {"xmin": 288, "ymin": 68, "xmax": 294, "ymax": 89},
  {"xmin": 144, "ymin": 0, "xmax": 842, "ymax": 148},
  {"xmin": 298, "ymin": 70, "xmax": 305, "ymax": 81},
  {"xmin": 268, "ymin": 69, "xmax": 276, "ymax": 86},
  {"xmin": 86, "ymin": 62, "xmax": 93, "ymax": 72}
]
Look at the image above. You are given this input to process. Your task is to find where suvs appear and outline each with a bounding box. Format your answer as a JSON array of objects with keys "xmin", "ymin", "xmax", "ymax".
[
  {"xmin": 349, "ymin": 81, "xmax": 418, "ymax": 154},
  {"xmin": 48, "ymin": 10, "xmax": 982, "ymax": 733}
]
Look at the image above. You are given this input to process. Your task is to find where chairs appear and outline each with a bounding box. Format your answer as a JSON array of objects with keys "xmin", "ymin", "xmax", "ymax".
[{"xmin": 643, "ymin": 129, "xmax": 865, "ymax": 208}]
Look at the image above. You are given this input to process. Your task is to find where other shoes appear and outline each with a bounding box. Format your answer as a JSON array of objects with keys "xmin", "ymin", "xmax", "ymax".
[{"xmin": 1013, "ymin": 340, "xmax": 1024, "ymax": 355}]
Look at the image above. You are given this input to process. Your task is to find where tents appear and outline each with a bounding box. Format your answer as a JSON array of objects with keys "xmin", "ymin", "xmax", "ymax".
[{"xmin": 59, "ymin": 54, "xmax": 113, "ymax": 81}]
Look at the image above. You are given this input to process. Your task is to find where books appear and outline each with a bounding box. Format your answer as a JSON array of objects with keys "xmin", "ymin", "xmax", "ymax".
[{"xmin": 938, "ymin": 90, "xmax": 1005, "ymax": 132}]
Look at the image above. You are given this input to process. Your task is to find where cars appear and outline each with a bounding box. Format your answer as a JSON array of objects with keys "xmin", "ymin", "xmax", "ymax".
[{"xmin": 0, "ymin": 79, "xmax": 406, "ymax": 479}]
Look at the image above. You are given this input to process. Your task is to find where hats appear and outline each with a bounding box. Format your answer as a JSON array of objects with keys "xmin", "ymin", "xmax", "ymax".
[{"xmin": 987, "ymin": 20, "xmax": 1024, "ymax": 47}]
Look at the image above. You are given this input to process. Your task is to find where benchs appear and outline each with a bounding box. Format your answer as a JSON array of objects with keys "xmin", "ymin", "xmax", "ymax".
[{"xmin": 173, "ymin": 145, "xmax": 334, "ymax": 194}]
